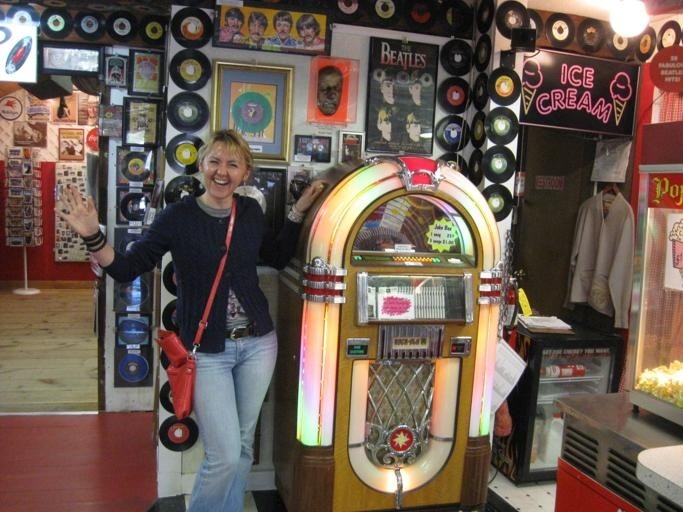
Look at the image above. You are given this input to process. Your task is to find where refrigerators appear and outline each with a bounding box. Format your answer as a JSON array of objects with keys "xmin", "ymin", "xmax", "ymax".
[{"xmin": 493, "ymin": 316, "xmax": 621, "ymax": 488}]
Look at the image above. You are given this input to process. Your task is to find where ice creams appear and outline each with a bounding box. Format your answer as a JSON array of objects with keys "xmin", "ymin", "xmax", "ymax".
[
  {"xmin": 522, "ymin": 60, "xmax": 543, "ymax": 114},
  {"xmin": 610, "ymin": 72, "xmax": 632, "ymax": 125}
]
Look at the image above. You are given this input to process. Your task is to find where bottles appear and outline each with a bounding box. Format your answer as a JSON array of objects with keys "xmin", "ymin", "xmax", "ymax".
[
  {"xmin": 540, "ymin": 364, "xmax": 585, "ymax": 376},
  {"xmin": 528, "ymin": 412, "xmax": 544, "ymax": 463}
]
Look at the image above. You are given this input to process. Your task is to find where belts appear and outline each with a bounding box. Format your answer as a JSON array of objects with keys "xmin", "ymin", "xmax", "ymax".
[{"xmin": 223, "ymin": 323, "xmax": 256, "ymax": 343}]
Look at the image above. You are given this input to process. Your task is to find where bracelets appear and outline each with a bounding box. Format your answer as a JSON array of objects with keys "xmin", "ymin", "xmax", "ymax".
[{"xmin": 79, "ymin": 229, "xmax": 107, "ymax": 251}]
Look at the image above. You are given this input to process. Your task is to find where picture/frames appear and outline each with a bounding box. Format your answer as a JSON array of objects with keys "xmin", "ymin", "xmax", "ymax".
[
  {"xmin": 0, "ymin": 96, "xmax": 22, "ymax": 120},
  {"xmin": 52, "ymin": 93, "xmax": 79, "ymax": 124},
  {"xmin": 122, "ymin": 97, "xmax": 161, "ymax": 144},
  {"xmin": 126, "ymin": 48, "xmax": 164, "ymax": 97},
  {"xmin": 364, "ymin": 34, "xmax": 440, "ymax": 154},
  {"xmin": 210, "ymin": 4, "xmax": 333, "ymax": 56},
  {"xmin": 209, "ymin": 57, "xmax": 295, "ymax": 168},
  {"xmin": 59, "ymin": 128, "xmax": 84, "ymax": 161}
]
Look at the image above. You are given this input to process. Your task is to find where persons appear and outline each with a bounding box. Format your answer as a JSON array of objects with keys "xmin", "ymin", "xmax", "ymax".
[{"xmin": 51, "ymin": 126, "xmax": 329, "ymax": 512}]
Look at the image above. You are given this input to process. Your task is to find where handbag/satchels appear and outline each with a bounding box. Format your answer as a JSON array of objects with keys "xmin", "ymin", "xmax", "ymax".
[{"xmin": 153, "ymin": 328, "xmax": 197, "ymax": 422}]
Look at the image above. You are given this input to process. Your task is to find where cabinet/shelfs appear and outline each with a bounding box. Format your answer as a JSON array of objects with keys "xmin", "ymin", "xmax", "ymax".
[{"xmin": 538, "ymin": 357, "xmax": 607, "ymax": 407}]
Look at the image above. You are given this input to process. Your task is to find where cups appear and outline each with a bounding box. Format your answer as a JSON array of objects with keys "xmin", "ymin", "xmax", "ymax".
[{"xmin": 671, "ymin": 240, "xmax": 681, "ymax": 268}]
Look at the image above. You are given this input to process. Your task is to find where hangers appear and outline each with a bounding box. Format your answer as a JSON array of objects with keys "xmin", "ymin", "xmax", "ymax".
[{"xmin": 598, "ymin": 179, "xmax": 620, "ymax": 199}]
